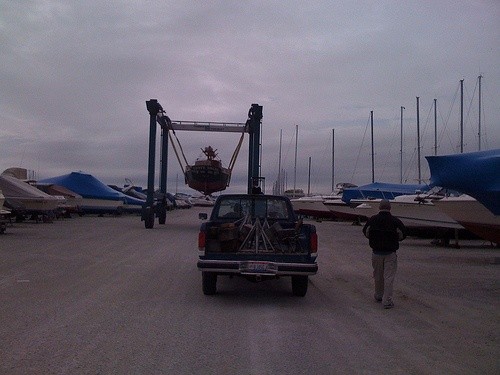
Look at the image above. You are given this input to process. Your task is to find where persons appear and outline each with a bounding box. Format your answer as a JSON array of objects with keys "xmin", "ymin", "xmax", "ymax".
[
  {"xmin": 224, "ymin": 203, "xmax": 245, "ymax": 218},
  {"xmin": 362, "ymin": 199, "xmax": 407, "ymax": 309}
]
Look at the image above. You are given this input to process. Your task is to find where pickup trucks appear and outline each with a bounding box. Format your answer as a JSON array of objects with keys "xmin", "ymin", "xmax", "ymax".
[{"xmin": 197, "ymin": 194, "xmax": 318, "ymax": 296}]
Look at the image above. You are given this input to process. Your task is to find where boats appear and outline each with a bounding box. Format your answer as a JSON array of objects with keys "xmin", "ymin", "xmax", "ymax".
[
  {"xmin": 285, "ymin": 150, "xmax": 500, "ymax": 248},
  {"xmin": 0, "ymin": 168, "xmax": 216, "ymax": 234},
  {"xmin": 184, "ymin": 146, "xmax": 231, "ymax": 196}
]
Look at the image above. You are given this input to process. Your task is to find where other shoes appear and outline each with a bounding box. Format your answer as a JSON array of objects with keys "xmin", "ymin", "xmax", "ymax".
[
  {"xmin": 375, "ymin": 297, "xmax": 382, "ymax": 302},
  {"xmin": 384, "ymin": 302, "xmax": 393, "ymax": 309}
]
